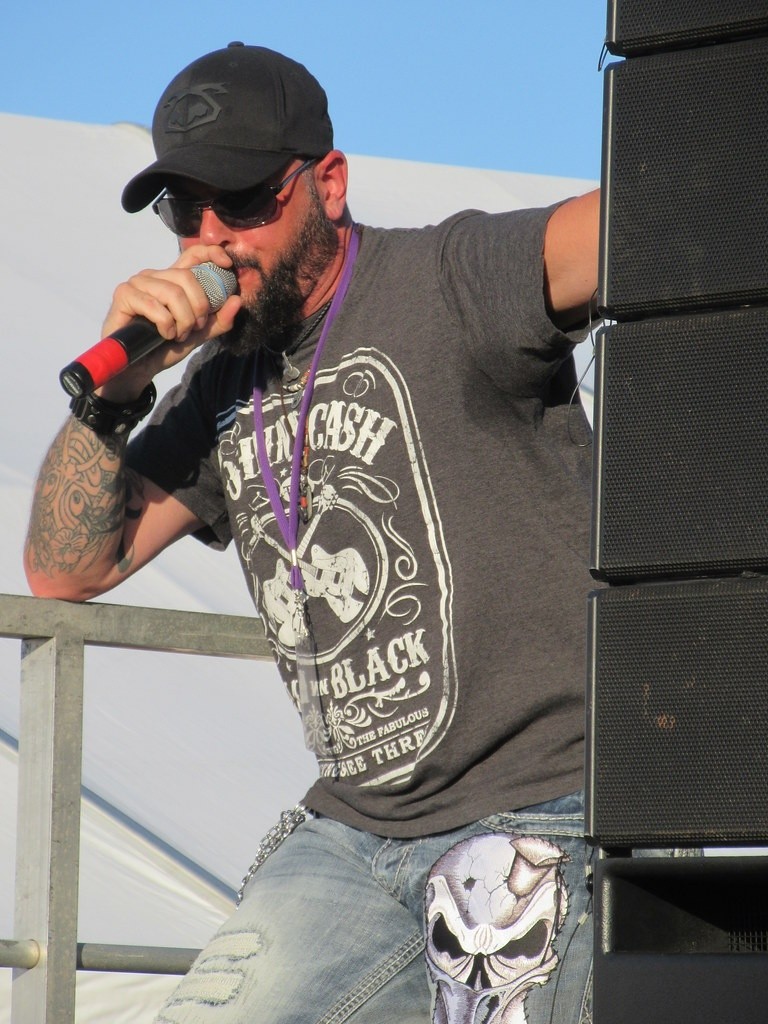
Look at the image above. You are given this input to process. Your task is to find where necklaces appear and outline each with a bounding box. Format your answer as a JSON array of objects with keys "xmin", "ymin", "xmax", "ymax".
[{"xmin": 253, "ymin": 227, "xmax": 359, "ymax": 759}]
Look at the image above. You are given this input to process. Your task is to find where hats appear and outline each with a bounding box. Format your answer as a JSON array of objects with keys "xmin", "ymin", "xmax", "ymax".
[{"xmin": 121, "ymin": 42, "xmax": 334, "ymax": 213}]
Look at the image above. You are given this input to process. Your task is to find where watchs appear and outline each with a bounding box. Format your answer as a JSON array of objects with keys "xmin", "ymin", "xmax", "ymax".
[{"xmin": 69, "ymin": 382, "xmax": 157, "ymax": 436}]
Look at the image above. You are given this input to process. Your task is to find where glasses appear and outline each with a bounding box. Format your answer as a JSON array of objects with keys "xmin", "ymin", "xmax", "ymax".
[{"xmin": 152, "ymin": 154, "xmax": 324, "ymax": 237}]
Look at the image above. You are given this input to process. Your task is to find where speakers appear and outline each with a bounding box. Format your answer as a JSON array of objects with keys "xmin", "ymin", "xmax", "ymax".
[{"xmin": 590, "ymin": 0, "xmax": 768, "ymax": 1024}]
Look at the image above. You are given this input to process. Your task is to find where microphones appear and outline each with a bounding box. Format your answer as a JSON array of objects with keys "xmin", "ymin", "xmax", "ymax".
[{"xmin": 60, "ymin": 262, "xmax": 238, "ymax": 398}]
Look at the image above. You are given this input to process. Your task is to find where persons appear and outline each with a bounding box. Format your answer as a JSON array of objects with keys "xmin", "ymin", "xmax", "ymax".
[{"xmin": 24, "ymin": 42, "xmax": 599, "ymax": 1024}]
